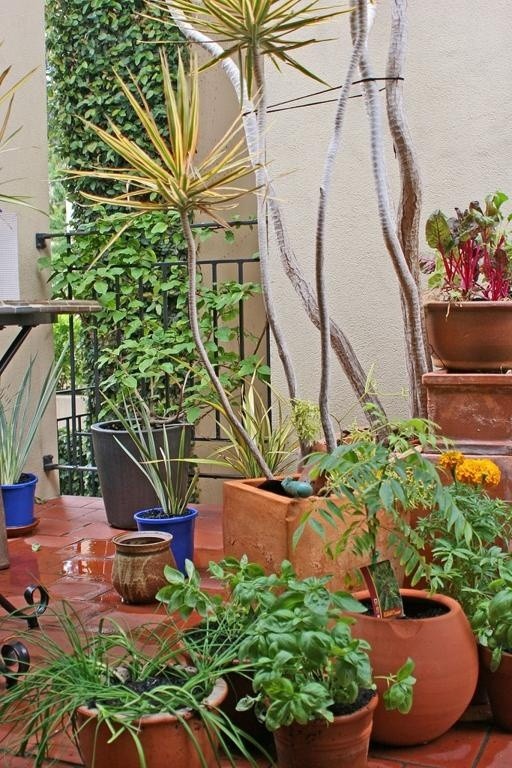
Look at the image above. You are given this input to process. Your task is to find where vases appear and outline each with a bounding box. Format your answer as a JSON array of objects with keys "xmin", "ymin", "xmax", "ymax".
[{"xmin": 111, "ymin": 531, "xmax": 179, "ymax": 607}]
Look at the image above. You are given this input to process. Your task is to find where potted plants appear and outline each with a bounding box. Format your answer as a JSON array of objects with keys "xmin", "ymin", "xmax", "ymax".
[
  {"xmin": 97, "ymin": 388, "xmax": 201, "ymax": 578},
  {"xmin": 1, "ymin": 345, "xmax": 70, "ymax": 539},
  {"xmin": 413, "ymin": 447, "xmax": 511, "ymax": 738},
  {"xmin": 417, "ymin": 191, "xmax": 511, "ymax": 374},
  {"xmin": 1, "ymin": 572, "xmax": 279, "ymax": 767},
  {"xmin": 153, "ymin": 553, "xmax": 270, "ymax": 756},
  {"xmin": 155, "ymin": 555, "xmax": 416, "ymax": 768},
  {"xmin": 60, "ymin": 1, "xmax": 414, "ymax": 592},
  {"xmin": 291, "ymin": 417, "xmax": 479, "ymax": 748},
  {"xmin": 34, "ymin": 202, "xmax": 270, "ymax": 532}
]
